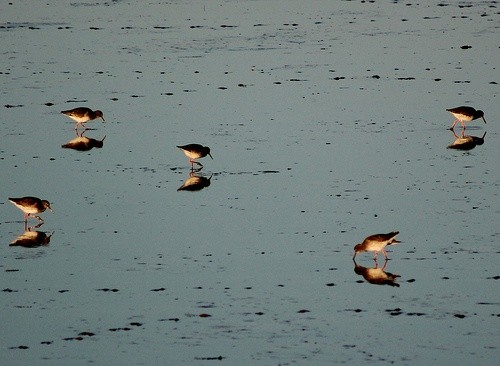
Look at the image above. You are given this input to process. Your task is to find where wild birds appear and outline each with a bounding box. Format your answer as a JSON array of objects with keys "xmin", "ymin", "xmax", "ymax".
[
  {"xmin": 445, "ymin": 129, "xmax": 486, "ymax": 150},
  {"xmin": 7, "ymin": 222, "xmax": 54, "ymax": 248},
  {"xmin": 8, "ymin": 196, "xmax": 52, "ymax": 222},
  {"xmin": 444, "ymin": 106, "xmax": 486, "ymax": 129},
  {"xmin": 177, "ymin": 167, "xmax": 213, "ymax": 191},
  {"xmin": 61, "ymin": 130, "xmax": 106, "ymax": 151},
  {"xmin": 352, "ymin": 231, "xmax": 401, "ymax": 259},
  {"xmin": 61, "ymin": 107, "xmax": 105, "ymax": 130},
  {"xmin": 353, "ymin": 259, "xmax": 401, "ymax": 287},
  {"xmin": 176, "ymin": 144, "xmax": 214, "ymax": 167}
]
